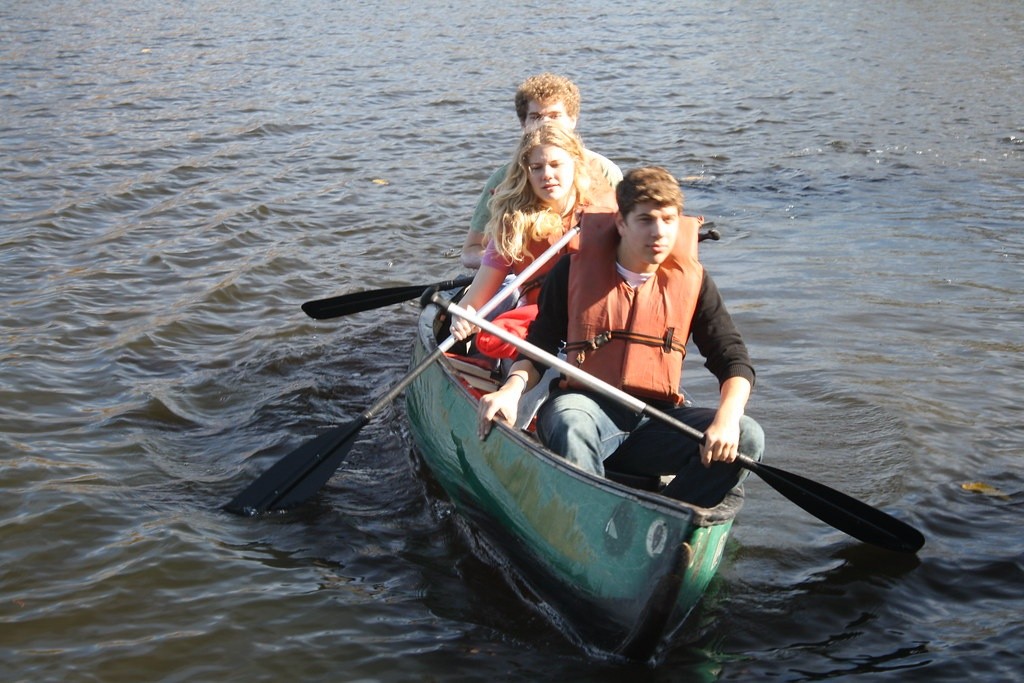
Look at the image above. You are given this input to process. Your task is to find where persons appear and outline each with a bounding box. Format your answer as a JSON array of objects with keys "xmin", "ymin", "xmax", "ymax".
[
  {"xmin": 449, "ymin": 123, "xmax": 614, "ymax": 431},
  {"xmin": 478, "ymin": 166, "xmax": 765, "ymax": 504},
  {"xmin": 463, "ymin": 74, "xmax": 625, "ymax": 323}
]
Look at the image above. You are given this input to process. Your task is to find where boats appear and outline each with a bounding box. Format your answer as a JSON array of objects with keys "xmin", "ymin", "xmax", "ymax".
[{"xmin": 405, "ymin": 274, "xmax": 745, "ymax": 663}]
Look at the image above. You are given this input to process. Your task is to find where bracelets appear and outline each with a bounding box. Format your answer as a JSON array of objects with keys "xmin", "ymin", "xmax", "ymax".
[{"xmin": 506, "ymin": 374, "xmax": 526, "ymax": 391}]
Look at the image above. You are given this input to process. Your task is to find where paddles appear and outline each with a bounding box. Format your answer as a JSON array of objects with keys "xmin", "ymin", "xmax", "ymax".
[
  {"xmin": 301, "ymin": 229, "xmax": 721, "ymax": 320},
  {"xmin": 419, "ymin": 285, "xmax": 924, "ymax": 555},
  {"xmin": 225, "ymin": 223, "xmax": 581, "ymax": 511}
]
